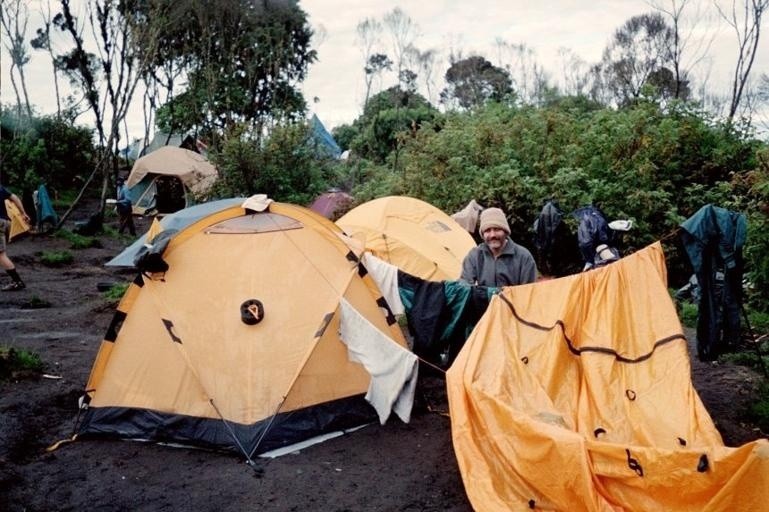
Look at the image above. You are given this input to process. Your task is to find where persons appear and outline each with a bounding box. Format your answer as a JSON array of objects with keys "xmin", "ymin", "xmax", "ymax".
[
  {"xmin": 0, "ymin": 181, "xmax": 30, "ymax": 292},
  {"xmin": 113, "ymin": 176, "xmax": 137, "ymax": 236},
  {"xmin": 458, "ymin": 206, "xmax": 537, "ymax": 286}
]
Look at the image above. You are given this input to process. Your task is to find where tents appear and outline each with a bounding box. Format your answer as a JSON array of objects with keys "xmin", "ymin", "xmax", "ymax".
[
  {"xmin": 102, "ymin": 197, "xmax": 251, "ymax": 268},
  {"xmin": 443, "ymin": 241, "xmax": 769, "ymax": 512},
  {"xmin": 114, "ymin": 111, "xmax": 353, "ymax": 220},
  {"xmin": 335, "ymin": 195, "xmax": 477, "ymax": 286},
  {"xmin": 75, "ymin": 192, "xmax": 434, "ymax": 466}
]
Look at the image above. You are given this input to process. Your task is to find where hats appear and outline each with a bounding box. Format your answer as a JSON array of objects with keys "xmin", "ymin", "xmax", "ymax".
[{"xmin": 479, "ymin": 207, "xmax": 511, "ymax": 236}]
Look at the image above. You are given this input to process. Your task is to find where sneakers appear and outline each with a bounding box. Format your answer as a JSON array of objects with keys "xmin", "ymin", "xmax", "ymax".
[{"xmin": 2, "ymin": 281, "xmax": 25, "ymax": 292}]
